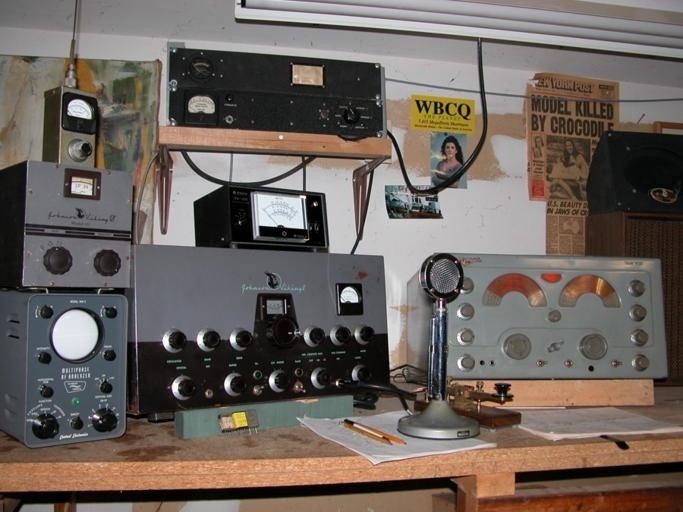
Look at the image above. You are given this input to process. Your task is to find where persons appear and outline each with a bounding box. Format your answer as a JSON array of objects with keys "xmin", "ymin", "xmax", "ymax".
[
  {"xmin": 430, "ymin": 136, "xmax": 464, "ymax": 189},
  {"xmin": 550, "ymin": 139, "xmax": 588, "ymax": 198},
  {"xmin": 533, "ymin": 136, "xmax": 544, "ymax": 162}
]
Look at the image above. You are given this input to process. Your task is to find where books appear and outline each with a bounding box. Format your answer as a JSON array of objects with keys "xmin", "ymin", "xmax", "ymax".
[
  {"xmin": 296, "ymin": 406, "xmax": 498, "ymax": 468},
  {"xmin": 508, "ymin": 403, "xmax": 683, "ymax": 443}
]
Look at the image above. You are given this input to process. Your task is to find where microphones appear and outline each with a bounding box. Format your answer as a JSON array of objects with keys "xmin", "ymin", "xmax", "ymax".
[{"xmin": 398, "ymin": 253, "xmax": 480, "ymax": 440}]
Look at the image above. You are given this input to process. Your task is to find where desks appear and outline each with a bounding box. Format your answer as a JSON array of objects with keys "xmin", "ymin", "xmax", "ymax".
[{"xmin": 1, "ymin": 386, "xmax": 683, "ymax": 512}]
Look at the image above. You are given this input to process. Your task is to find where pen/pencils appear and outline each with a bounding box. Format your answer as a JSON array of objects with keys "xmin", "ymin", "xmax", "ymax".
[{"xmin": 339, "ymin": 419, "xmax": 408, "ymax": 446}]
